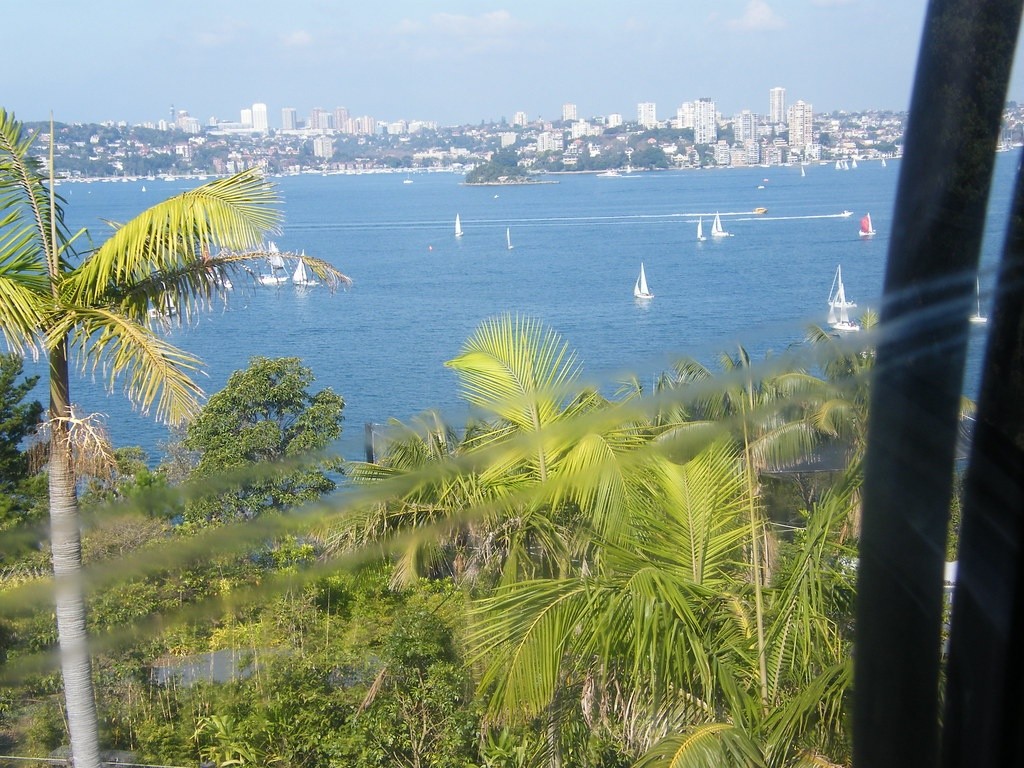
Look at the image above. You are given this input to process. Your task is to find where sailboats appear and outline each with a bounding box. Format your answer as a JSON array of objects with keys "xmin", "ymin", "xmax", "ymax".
[
  {"xmin": 834, "ymin": 158, "xmax": 858, "ymax": 172},
  {"xmin": 710, "ymin": 210, "xmax": 736, "ymax": 238},
  {"xmin": 505, "ymin": 227, "xmax": 514, "ymax": 252},
  {"xmin": 257, "ymin": 241, "xmax": 291, "ymax": 285},
  {"xmin": 881, "ymin": 158, "xmax": 886, "ymax": 168},
  {"xmin": 633, "ymin": 262, "xmax": 655, "ymax": 299},
  {"xmin": 827, "ymin": 282, "xmax": 860, "ymax": 332},
  {"xmin": 293, "ymin": 249, "xmax": 319, "ymax": 287},
  {"xmin": 859, "ymin": 212, "xmax": 877, "ymax": 238},
  {"xmin": 967, "ymin": 276, "xmax": 988, "ymax": 323},
  {"xmin": 697, "ymin": 215, "xmax": 708, "ymax": 242},
  {"xmin": 801, "ymin": 165, "xmax": 806, "ymax": 177},
  {"xmin": 510, "ymin": 250, "xmax": 510, "ymax": 253},
  {"xmin": 827, "ymin": 263, "xmax": 859, "ymax": 308},
  {"xmin": 454, "ymin": 213, "xmax": 464, "ymax": 237}
]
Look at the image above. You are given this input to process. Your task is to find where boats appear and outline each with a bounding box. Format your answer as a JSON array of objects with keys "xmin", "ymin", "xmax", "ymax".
[
  {"xmin": 596, "ymin": 170, "xmax": 623, "ymax": 177},
  {"xmin": 148, "ymin": 280, "xmax": 182, "ymax": 318},
  {"xmin": 995, "ymin": 139, "xmax": 1015, "ymax": 153},
  {"xmin": 403, "ymin": 179, "xmax": 414, "ymax": 184},
  {"xmin": 758, "ymin": 178, "xmax": 769, "ymax": 189},
  {"xmin": 753, "ymin": 207, "xmax": 768, "ymax": 214},
  {"xmin": 696, "ymin": 161, "xmax": 828, "ymax": 170},
  {"xmin": 841, "ymin": 210, "xmax": 855, "ymax": 217},
  {"xmin": 198, "ymin": 231, "xmax": 232, "ymax": 289}
]
